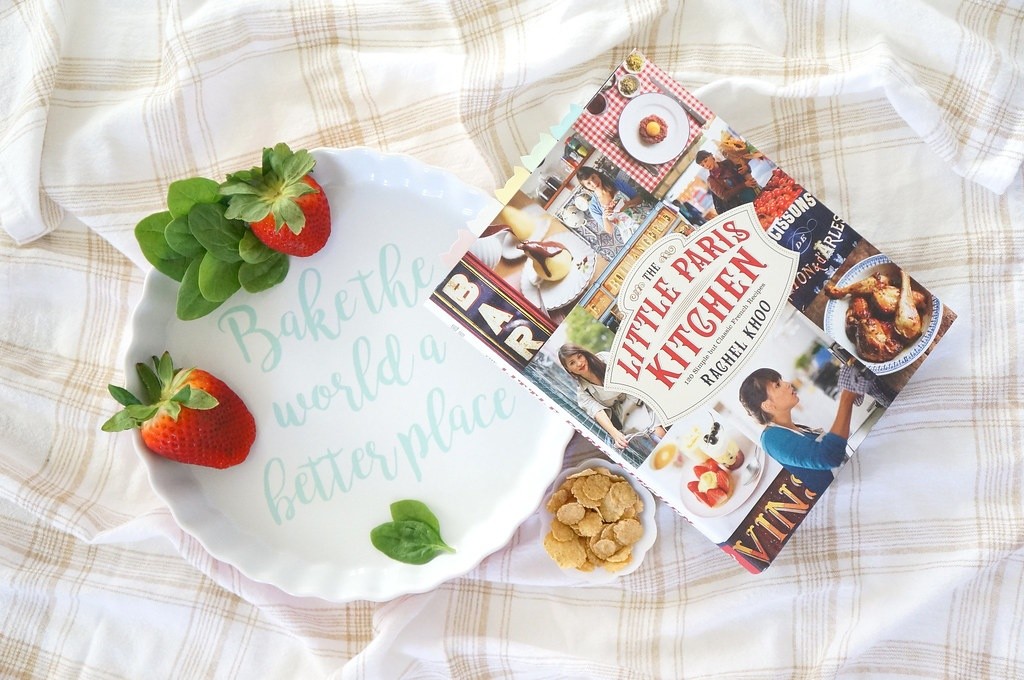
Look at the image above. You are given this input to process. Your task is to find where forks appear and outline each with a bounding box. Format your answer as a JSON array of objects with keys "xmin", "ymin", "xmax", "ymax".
[{"xmin": 603, "ymin": 127, "xmax": 659, "ymax": 177}]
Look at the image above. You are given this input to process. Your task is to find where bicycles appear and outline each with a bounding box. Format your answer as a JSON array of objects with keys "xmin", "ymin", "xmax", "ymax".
[{"xmin": 613, "ymin": 410, "xmax": 662, "ymax": 450}]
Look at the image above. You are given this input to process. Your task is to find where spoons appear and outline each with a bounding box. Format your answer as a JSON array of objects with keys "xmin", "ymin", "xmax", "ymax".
[{"xmin": 527, "ymin": 271, "xmax": 552, "ymax": 319}]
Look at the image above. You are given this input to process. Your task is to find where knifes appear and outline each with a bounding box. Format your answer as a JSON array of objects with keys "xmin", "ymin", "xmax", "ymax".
[{"xmin": 649, "ymin": 75, "xmax": 707, "ymax": 125}]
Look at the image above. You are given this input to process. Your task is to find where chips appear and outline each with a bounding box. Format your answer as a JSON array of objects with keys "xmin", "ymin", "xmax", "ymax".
[{"xmin": 544, "ymin": 461, "xmax": 643, "ymax": 575}]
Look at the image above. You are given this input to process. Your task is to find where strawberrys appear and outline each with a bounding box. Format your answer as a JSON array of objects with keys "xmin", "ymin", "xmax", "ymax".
[
  {"xmin": 221, "ymin": 146, "xmax": 330, "ymax": 257},
  {"xmin": 100, "ymin": 350, "xmax": 255, "ymax": 470}
]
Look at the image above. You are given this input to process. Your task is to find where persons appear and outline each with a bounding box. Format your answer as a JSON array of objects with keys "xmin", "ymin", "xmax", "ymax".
[
  {"xmin": 695, "ymin": 149, "xmax": 756, "ymax": 201},
  {"xmin": 558, "ymin": 342, "xmax": 667, "ymax": 450},
  {"xmin": 574, "ymin": 166, "xmax": 654, "ymax": 246},
  {"xmin": 738, "ymin": 367, "xmax": 858, "ymax": 496}
]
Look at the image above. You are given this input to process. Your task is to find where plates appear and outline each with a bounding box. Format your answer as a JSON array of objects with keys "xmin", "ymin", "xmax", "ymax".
[
  {"xmin": 120, "ymin": 145, "xmax": 577, "ymax": 605},
  {"xmin": 618, "ymin": 93, "xmax": 690, "ymax": 165},
  {"xmin": 500, "ymin": 209, "xmax": 551, "ymax": 261},
  {"xmin": 823, "ymin": 253, "xmax": 944, "ymax": 377},
  {"xmin": 520, "ymin": 232, "xmax": 597, "ymax": 311},
  {"xmin": 676, "ymin": 442, "xmax": 767, "ymax": 519}
]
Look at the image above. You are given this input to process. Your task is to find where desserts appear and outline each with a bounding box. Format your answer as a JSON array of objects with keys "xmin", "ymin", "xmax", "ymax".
[
  {"xmin": 638, "ymin": 115, "xmax": 668, "ymax": 144},
  {"xmin": 680, "ymin": 427, "xmax": 717, "ymax": 466},
  {"xmin": 516, "ymin": 241, "xmax": 573, "ymax": 280},
  {"xmin": 688, "ymin": 460, "xmax": 732, "ymax": 509},
  {"xmin": 700, "ymin": 424, "xmax": 745, "ymax": 467},
  {"xmin": 500, "ymin": 206, "xmax": 534, "ymax": 240}
]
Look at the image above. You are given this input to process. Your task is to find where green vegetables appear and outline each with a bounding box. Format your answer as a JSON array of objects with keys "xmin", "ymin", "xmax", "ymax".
[
  {"xmin": 369, "ymin": 501, "xmax": 457, "ymax": 565},
  {"xmin": 135, "ymin": 177, "xmax": 290, "ymax": 321}
]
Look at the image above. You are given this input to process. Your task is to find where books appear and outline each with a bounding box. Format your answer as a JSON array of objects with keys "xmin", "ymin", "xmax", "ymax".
[{"xmin": 427, "ymin": 46, "xmax": 961, "ymax": 576}]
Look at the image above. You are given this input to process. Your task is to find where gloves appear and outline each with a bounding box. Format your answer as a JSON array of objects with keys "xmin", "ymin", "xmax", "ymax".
[{"xmin": 837, "ymin": 359, "xmax": 876, "ymax": 406}]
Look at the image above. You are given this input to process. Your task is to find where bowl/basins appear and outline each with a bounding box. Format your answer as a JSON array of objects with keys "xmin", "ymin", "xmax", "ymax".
[
  {"xmin": 613, "ymin": 197, "xmax": 625, "ymax": 213},
  {"xmin": 538, "ymin": 458, "xmax": 658, "ymax": 587}
]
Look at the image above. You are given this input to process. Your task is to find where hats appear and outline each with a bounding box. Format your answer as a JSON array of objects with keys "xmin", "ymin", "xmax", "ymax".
[{"xmin": 695, "ymin": 150, "xmax": 713, "ymax": 165}]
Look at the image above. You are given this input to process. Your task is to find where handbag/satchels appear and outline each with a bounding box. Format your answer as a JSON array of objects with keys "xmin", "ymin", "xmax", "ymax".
[{"xmin": 709, "ymin": 175, "xmax": 730, "ymax": 215}]
